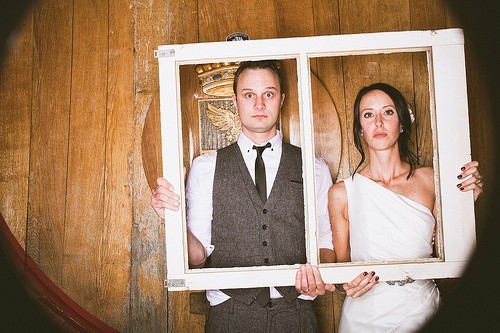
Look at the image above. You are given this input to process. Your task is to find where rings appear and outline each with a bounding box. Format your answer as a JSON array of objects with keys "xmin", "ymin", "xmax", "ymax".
[
  {"xmin": 477, "ymin": 177, "xmax": 483, "ymax": 184},
  {"xmin": 303, "ymin": 289, "xmax": 310, "ymax": 293},
  {"xmin": 153, "ymin": 186, "xmax": 160, "ymax": 193}
]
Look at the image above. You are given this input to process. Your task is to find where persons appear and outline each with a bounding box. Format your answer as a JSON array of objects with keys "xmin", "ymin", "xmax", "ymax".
[
  {"xmin": 149, "ymin": 59, "xmax": 337, "ymax": 333},
  {"xmin": 326, "ymin": 82, "xmax": 485, "ymax": 333}
]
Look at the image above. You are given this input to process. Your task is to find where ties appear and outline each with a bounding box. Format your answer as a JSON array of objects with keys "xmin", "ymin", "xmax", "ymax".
[{"xmin": 253, "ymin": 142, "xmax": 270, "ymax": 308}]
